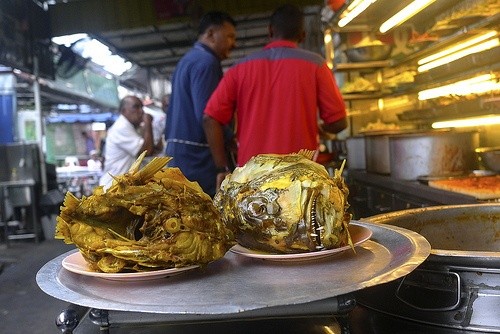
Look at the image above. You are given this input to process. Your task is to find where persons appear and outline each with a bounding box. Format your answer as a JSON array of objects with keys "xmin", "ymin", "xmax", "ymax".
[
  {"xmin": 99, "ymin": 95, "xmax": 170, "ymax": 193},
  {"xmin": 166, "ymin": 10, "xmax": 236, "ymax": 200},
  {"xmin": 81, "ymin": 131, "xmax": 96, "ymax": 155},
  {"xmin": 201, "ymin": 3, "xmax": 348, "ymax": 194}
]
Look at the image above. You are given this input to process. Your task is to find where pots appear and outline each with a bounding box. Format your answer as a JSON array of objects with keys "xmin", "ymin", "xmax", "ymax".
[
  {"xmin": 344, "ymin": 126, "xmax": 482, "ymax": 181},
  {"xmin": 340, "ymin": 202, "xmax": 500, "ymax": 334}
]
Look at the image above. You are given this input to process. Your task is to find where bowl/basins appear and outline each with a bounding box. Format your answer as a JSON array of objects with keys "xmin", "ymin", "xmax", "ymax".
[
  {"xmin": 342, "ymin": 45, "xmax": 395, "ymax": 62},
  {"xmin": 473, "ymin": 147, "xmax": 500, "ymax": 174}
]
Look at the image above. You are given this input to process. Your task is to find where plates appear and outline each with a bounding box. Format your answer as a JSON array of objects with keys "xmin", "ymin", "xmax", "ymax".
[
  {"xmin": 61, "ymin": 250, "xmax": 217, "ymax": 283},
  {"xmin": 230, "ymin": 223, "xmax": 373, "ymax": 261}
]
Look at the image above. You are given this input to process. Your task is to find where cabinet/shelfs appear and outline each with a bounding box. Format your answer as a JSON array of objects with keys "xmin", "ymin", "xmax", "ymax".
[
  {"xmin": 351, "ymin": 183, "xmax": 432, "ymax": 221},
  {"xmin": 324, "ymin": 0, "xmax": 500, "ymax": 139}
]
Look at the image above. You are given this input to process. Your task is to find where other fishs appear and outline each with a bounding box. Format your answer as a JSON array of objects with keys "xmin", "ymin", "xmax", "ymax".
[
  {"xmin": 54, "ymin": 151, "xmax": 225, "ymax": 272},
  {"xmin": 211, "ymin": 148, "xmax": 351, "ymax": 252}
]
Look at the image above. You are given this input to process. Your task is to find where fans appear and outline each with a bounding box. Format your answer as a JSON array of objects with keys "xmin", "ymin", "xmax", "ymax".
[{"xmin": 51, "ymin": 37, "xmax": 91, "ymax": 79}]
